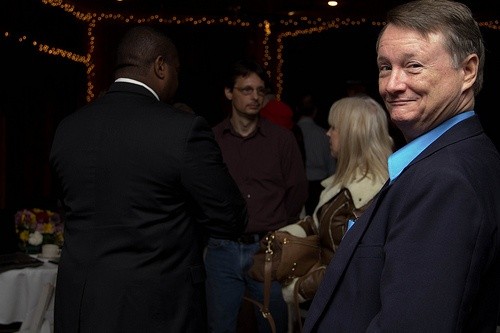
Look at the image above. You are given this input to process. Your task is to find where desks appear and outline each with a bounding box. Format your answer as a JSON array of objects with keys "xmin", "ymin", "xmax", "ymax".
[{"xmin": 0, "ymin": 250, "xmax": 62, "ymax": 333}]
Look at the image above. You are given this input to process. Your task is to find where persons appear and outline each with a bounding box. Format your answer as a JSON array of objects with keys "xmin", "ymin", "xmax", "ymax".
[
  {"xmin": 301, "ymin": 0, "xmax": 500, "ymax": 333},
  {"xmin": 204, "ymin": 60, "xmax": 309, "ymax": 333},
  {"xmin": 49, "ymin": 25, "xmax": 249, "ymax": 333},
  {"xmin": 297, "ymin": 95, "xmax": 336, "ymax": 217},
  {"xmin": 281, "ymin": 97, "xmax": 393, "ymax": 304},
  {"xmin": 258, "ymin": 74, "xmax": 307, "ymax": 169}
]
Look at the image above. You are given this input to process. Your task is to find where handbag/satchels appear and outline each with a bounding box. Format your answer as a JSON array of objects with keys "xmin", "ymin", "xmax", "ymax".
[{"xmin": 246, "ymin": 231, "xmax": 335, "ymax": 282}]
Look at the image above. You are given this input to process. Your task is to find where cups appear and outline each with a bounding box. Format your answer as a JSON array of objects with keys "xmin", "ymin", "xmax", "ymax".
[{"xmin": 41, "ymin": 244, "xmax": 62, "ymax": 257}]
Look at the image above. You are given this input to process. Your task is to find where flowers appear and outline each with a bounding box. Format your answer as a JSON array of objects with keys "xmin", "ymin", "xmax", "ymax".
[{"xmin": 13, "ymin": 206, "xmax": 63, "ymax": 245}]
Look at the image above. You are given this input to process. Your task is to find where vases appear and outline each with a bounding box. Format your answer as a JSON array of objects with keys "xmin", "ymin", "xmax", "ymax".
[{"xmin": 19, "ymin": 237, "xmax": 52, "ymax": 255}]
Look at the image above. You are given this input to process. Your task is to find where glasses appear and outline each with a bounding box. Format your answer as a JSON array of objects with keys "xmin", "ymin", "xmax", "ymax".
[{"xmin": 235, "ymin": 86, "xmax": 268, "ymax": 97}]
